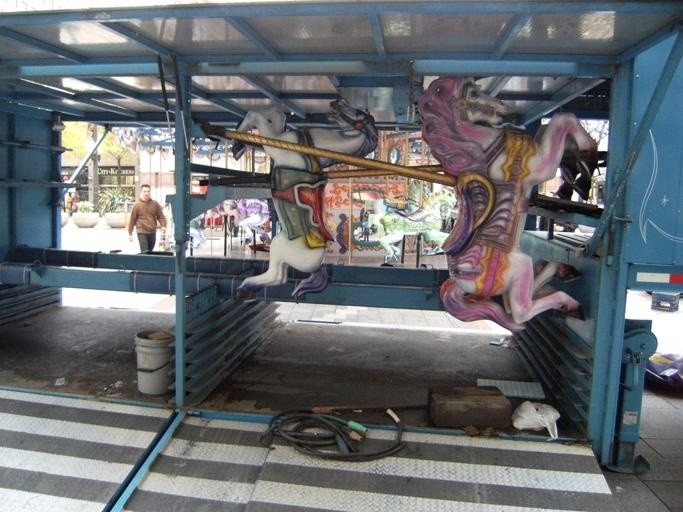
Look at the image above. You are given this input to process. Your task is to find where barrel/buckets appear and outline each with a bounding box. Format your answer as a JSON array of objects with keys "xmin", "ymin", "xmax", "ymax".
[{"xmin": 131, "ymin": 329, "xmax": 175, "ymax": 395}]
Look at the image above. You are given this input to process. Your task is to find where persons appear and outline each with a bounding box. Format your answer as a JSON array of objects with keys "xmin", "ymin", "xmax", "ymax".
[{"xmin": 129, "ymin": 185, "xmax": 166, "ymax": 254}]
